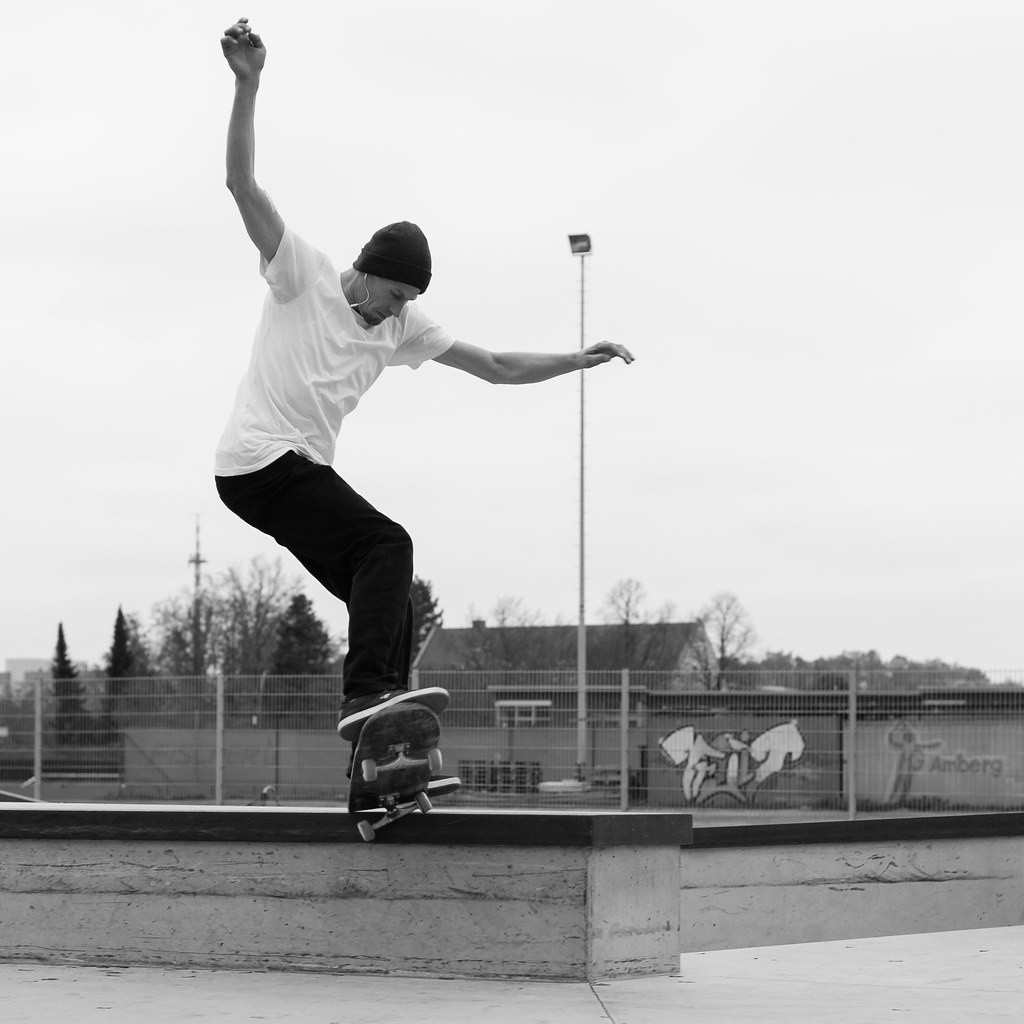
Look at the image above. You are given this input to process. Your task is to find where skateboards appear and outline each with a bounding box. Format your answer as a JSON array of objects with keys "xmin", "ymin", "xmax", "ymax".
[{"xmin": 348, "ymin": 703, "xmax": 445, "ymax": 842}]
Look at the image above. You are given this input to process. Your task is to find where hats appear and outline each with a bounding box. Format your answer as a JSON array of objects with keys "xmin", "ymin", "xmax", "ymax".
[{"xmin": 353, "ymin": 221, "xmax": 432, "ymax": 295}]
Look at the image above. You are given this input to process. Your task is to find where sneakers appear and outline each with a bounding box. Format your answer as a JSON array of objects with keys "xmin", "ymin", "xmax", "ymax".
[
  {"xmin": 338, "ymin": 687, "xmax": 450, "ymax": 741},
  {"xmin": 426, "ymin": 775, "xmax": 462, "ymax": 797}
]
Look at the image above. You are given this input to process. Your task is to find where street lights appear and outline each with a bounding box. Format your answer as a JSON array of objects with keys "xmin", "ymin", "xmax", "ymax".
[{"xmin": 568, "ymin": 234, "xmax": 591, "ymax": 782}]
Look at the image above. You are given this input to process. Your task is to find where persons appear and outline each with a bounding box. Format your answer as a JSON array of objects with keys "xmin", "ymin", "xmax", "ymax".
[{"xmin": 215, "ymin": 19, "xmax": 634, "ymax": 799}]
